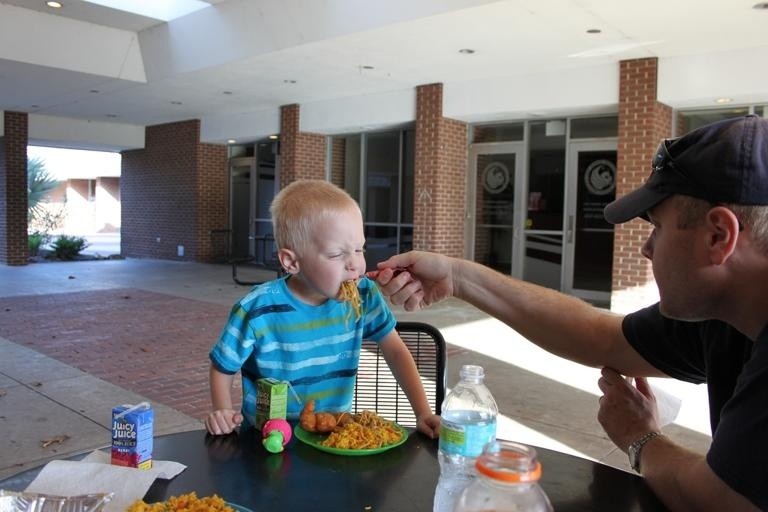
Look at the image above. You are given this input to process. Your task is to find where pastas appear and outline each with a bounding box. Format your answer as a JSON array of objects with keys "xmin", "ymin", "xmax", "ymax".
[
  {"xmin": 322, "ymin": 410, "xmax": 401, "ymax": 449},
  {"xmin": 341, "ymin": 279, "xmax": 365, "ymax": 328}
]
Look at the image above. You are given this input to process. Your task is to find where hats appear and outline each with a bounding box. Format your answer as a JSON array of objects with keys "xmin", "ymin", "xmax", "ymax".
[{"xmin": 603, "ymin": 114, "xmax": 768, "ymax": 228}]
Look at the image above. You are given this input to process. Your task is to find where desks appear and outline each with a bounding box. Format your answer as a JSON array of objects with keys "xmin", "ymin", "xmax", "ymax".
[{"xmin": 248, "ymin": 235, "xmax": 284, "ymax": 279}]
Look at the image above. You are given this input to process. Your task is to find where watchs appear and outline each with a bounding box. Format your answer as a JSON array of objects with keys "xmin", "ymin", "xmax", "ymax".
[{"xmin": 627, "ymin": 431, "xmax": 659, "ymax": 473}]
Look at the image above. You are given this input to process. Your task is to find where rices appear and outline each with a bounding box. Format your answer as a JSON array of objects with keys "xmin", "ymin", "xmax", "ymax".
[{"xmin": 126, "ymin": 491, "xmax": 238, "ymax": 512}]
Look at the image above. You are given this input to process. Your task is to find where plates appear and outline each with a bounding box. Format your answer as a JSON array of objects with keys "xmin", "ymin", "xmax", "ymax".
[{"xmin": 295, "ymin": 415, "xmax": 408, "ymax": 457}]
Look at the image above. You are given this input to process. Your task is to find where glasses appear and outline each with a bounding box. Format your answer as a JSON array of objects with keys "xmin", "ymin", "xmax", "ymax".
[{"xmin": 650, "ymin": 137, "xmax": 744, "ymax": 233}]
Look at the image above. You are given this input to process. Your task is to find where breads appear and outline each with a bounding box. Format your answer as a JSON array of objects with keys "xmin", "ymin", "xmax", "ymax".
[{"xmin": 302, "ymin": 399, "xmax": 350, "ymax": 433}]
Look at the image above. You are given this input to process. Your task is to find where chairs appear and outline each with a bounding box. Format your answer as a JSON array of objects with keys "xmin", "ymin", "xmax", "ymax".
[
  {"xmin": 352, "ymin": 321, "xmax": 447, "ymax": 428},
  {"xmin": 210, "ymin": 229, "xmax": 255, "ymax": 284},
  {"xmin": 262, "ymin": 233, "xmax": 286, "ymax": 278}
]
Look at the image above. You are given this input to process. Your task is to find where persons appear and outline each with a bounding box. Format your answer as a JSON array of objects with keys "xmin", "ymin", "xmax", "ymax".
[
  {"xmin": 374, "ymin": 116, "xmax": 766, "ymax": 509},
  {"xmin": 205, "ymin": 178, "xmax": 443, "ymax": 439}
]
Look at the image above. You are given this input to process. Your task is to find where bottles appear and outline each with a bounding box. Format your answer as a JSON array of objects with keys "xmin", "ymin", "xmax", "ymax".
[{"xmin": 434, "ymin": 363, "xmax": 555, "ymax": 511}]
